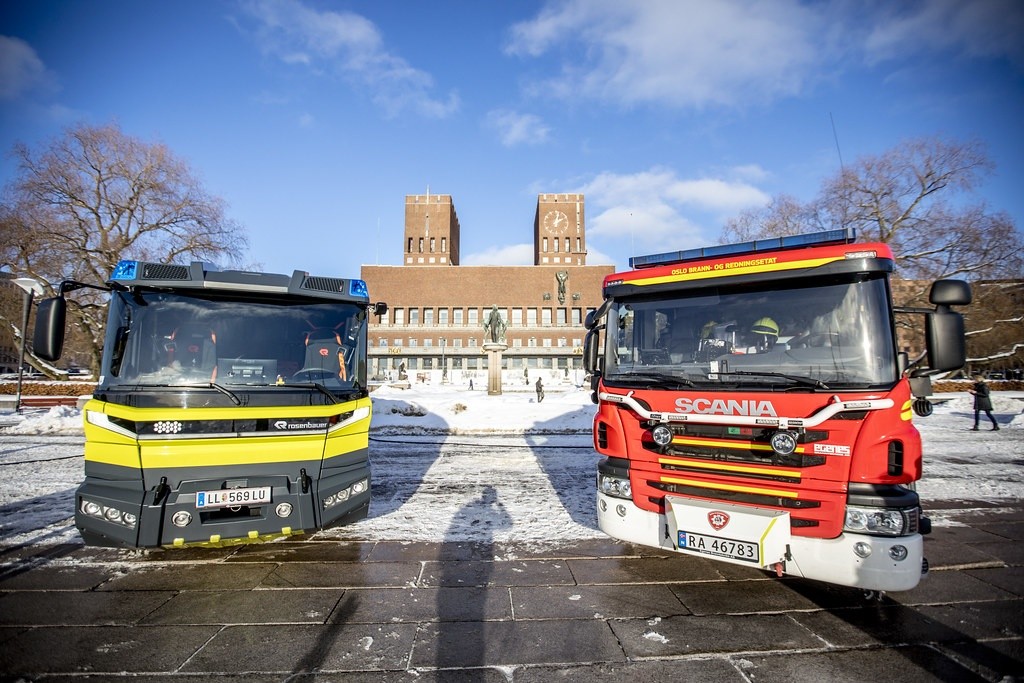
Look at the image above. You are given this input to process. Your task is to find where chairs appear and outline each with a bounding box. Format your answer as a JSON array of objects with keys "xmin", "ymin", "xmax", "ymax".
[
  {"xmin": 167, "ymin": 322, "xmax": 217, "ymax": 383},
  {"xmin": 301, "ymin": 327, "xmax": 349, "ymax": 381},
  {"xmin": 667, "ymin": 316, "xmax": 696, "ymax": 363},
  {"xmin": 808, "ymin": 303, "xmax": 847, "ymax": 347}
]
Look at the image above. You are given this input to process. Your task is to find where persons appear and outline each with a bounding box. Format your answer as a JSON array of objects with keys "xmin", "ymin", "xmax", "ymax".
[
  {"xmin": 556, "ymin": 269, "xmax": 569, "ymax": 302},
  {"xmin": 483, "ymin": 304, "xmax": 507, "ymax": 342},
  {"xmin": 421, "ymin": 367, "xmax": 545, "ymax": 403},
  {"xmin": 967, "ymin": 375, "xmax": 999, "ymax": 431}
]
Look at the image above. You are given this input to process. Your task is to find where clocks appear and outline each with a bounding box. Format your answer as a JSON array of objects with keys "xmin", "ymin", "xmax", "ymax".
[{"xmin": 543, "ymin": 209, "xmax": 569, "ymax": 235}]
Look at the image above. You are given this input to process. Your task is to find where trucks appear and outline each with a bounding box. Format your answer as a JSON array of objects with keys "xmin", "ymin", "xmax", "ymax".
[
  {"xmin": 583, "ymin": 227, "xmax": 971, "ymax": 593},
  {"xmin": 31, "ymin": 257, "xmax": 388, "ymax": 550}
]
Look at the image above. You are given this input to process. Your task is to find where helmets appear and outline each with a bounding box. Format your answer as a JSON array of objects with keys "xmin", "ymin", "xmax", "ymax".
[{"xmin": 749, "ymin": 316, "xmax": 779, "ymax": 341}]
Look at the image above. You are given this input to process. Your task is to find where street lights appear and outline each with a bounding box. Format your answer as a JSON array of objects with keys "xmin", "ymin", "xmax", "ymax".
[{"xmin": 8, "ymin": 278, "xmax": 47, "ymax": 412}]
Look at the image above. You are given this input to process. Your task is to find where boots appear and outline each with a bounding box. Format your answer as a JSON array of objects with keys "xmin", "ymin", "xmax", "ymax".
[
  {"xmin": 970, "ymin": 425, "xmax": 978, "ymax": 430},
  {"xmin": 991, "ymin": 423, "xmax": 1000, "ymax": 430}
]
